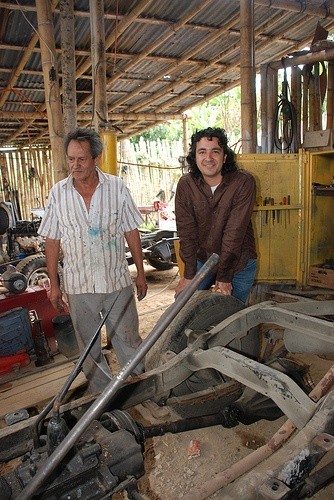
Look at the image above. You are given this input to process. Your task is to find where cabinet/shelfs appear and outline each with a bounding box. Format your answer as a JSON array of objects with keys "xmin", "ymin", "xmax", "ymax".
[{"xmin": 236, "ymin": 148, "xmax": 334, "ymax": 290}]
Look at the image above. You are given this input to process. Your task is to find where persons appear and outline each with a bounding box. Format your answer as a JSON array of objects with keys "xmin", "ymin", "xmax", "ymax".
[
  {"xmin": 36, "ymin": 127, "xmax": 148, "ymax": 393},
  {"xmin": 175, "ymin": 128, "xmax": 257, "ymax": 303}
]
